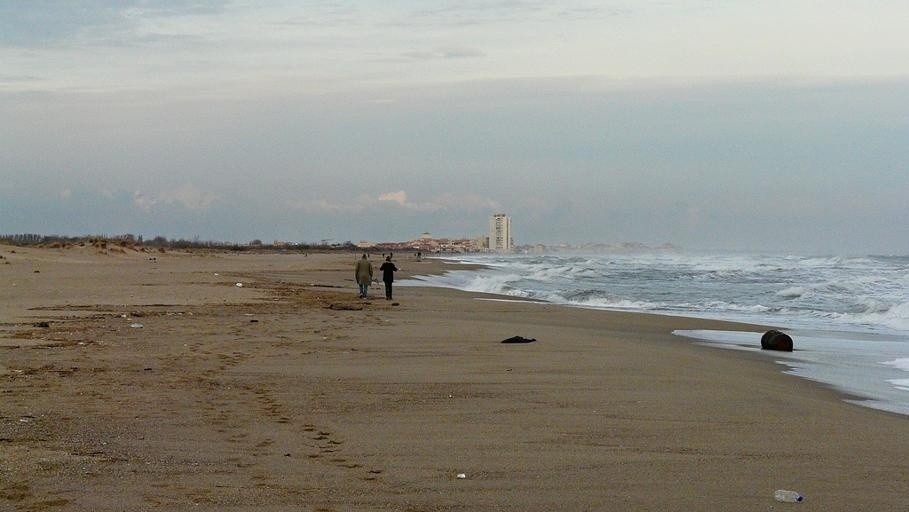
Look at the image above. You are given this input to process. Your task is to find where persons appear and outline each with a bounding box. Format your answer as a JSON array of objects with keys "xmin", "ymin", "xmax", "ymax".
[
  {"xmin": 380, "ymin": 256, "xmax": 397, "ymax": 300},
  {"xmin": 356, "ymin": 253, "xmax": 373, "ymax": 298}
]
